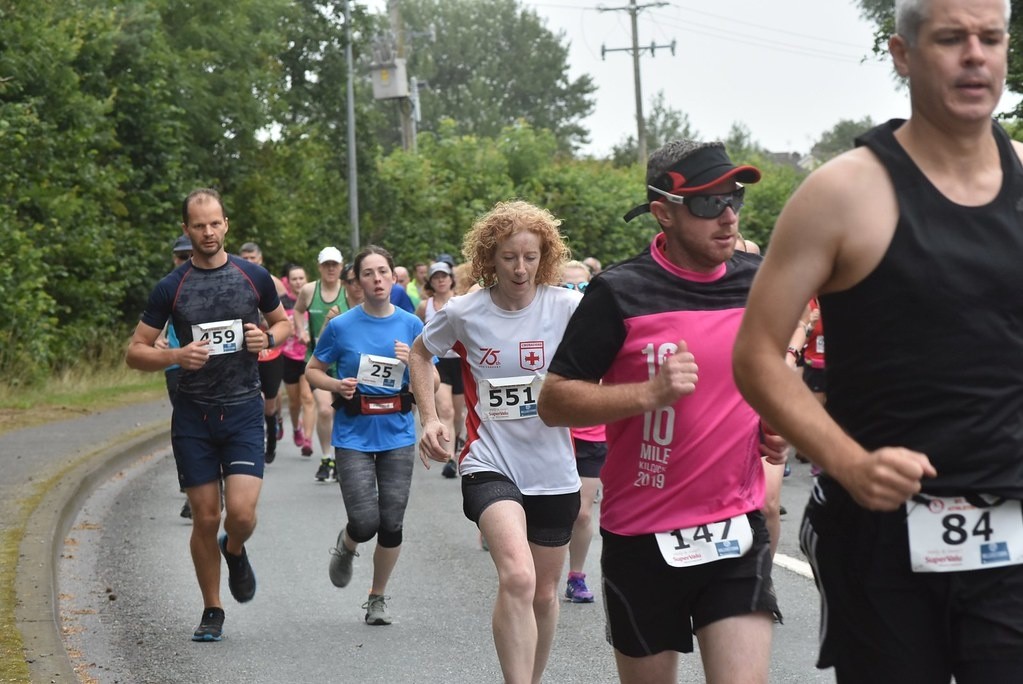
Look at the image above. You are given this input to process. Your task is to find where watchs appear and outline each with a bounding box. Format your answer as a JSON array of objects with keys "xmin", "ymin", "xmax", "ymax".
[
  {"xmin": 787, "ymin": 347, "xmax": 805, "ymax": 368},
  {"xmin": 265, "ymin": 331, "xmax": 274, "ymax": 349}
]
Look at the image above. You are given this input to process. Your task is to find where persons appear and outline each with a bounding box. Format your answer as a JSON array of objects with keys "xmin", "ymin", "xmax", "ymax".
[
  {"xmin": 155, "ymin": 232, "xmax": 828, "ymax": 520},
  {"xmin": 407, "ymin": 200, "xmax": 587, "ymax": 684},
  {"xmin": 125, "ymin": 188, "xmax": 292, "ymax": 642},
  {"xmin": 546, "ymin": 260, "xmax": 604, "ymax": 603},
  {"xmin": 538, "ymin": 138, "xmax": 789, "ymax": 684},
  {"xmin": 731, "ymin": 0, "xmax": 1023, "ymax": 684},
  {"xmin": 304, "ymin": 245, "xmax": 442, "ymax": 627}
]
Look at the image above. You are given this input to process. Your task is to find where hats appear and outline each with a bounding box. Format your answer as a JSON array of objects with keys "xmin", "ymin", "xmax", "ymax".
[
  {"xmin": 435, "ymin": 254, "xmax": 454, "ymax": 265},
  {"xmin": 172, "ymin": 235, "xmax": 193, "ymax": 251},
  {"xmin": 428, "ymin": 262, "xmax": 451, "ymax": 279},
  {"xmin": 623, "ymin": 147, "xmax": 761, "ymax": 223},
  {"xmin": 318, "ymin": 247, "xmax": 343, "ymax": 264}
]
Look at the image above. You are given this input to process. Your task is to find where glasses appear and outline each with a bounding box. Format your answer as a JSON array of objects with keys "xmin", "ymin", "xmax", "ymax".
[
  {"xmin": 345, "ymin": 278, "xmax": 358, "ymax": 285},
  {"xmin": 175, "ymin": 254, "xmax": 193, "ymax": 260},
  {"xmin": 562, "ymin": 282, "xmax": 589, "ymax": 293},
  {"xmin": 648, "ymin": 182, "xmax": 745, "ymax": 219}
]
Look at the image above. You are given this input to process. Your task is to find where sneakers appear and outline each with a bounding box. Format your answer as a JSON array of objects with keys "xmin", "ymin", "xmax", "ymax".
[
  {"xmin": 265, "ymin": 437, "xmax": 277, "ymax": 463},
  {"xmin": 293, "ymin": 419, "xmax": 304, "ymax": 447},
  {"xmin": 442, "ymin": 459, "xmax": 457, "ymax": 477},
  {"xmin": 455, "ymin": 436, "xmax": 459, "ymax": 453},
  {"xmin": 191, "ymin": 607, "xmax": 225, "ymax": 641},
  {"xmin": 361, "ymin": 595, "xmax": 392, "ymax": 625},
  {"xmin": 219, "ymin": 533, "xmax": 256, "ymax": 603},
  {"xmin": 314, "ymin": 458, "xmax": 335, "ymax": 481},
  {"xmin": 564, "ymin": 572, "xmax": 594, "ymax": 602},
  {"xmin": 328, "ymin": 529, "xmax": 360, "ymax": 588},
  {"xmin": 302, "ymin": 439, "xmax": 313, "ymax": 456}
]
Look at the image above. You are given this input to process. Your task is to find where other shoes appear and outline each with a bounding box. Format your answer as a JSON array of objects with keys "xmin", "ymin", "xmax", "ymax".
[
  {"xmin": 594, "ymin": 490, "xmax": 601, "ymax": 503},
  {"xmin": 180, "ymin": 499, "xmax": 192, "ymax": 519},
  {"xmin": 459, "ymin": 440, "xmax": 465, "ymax": 451},
  {"xmin": 220, "ymin": 480, "xmax": 224, "ymax": 514},
  {"xmin": 784, "ymin": 454, "xmax": 823, "ymax": 477},
  {"xmin": 780, "ymin": 505, "xmax": 787, "ymax": 515},
  {"xmin": 277, "ymin": 418, "xmax": 283, "ymax": 439},
  {"xmin": 481, "ymin": 532, "xmax": 489, "ymax": 552}
]
